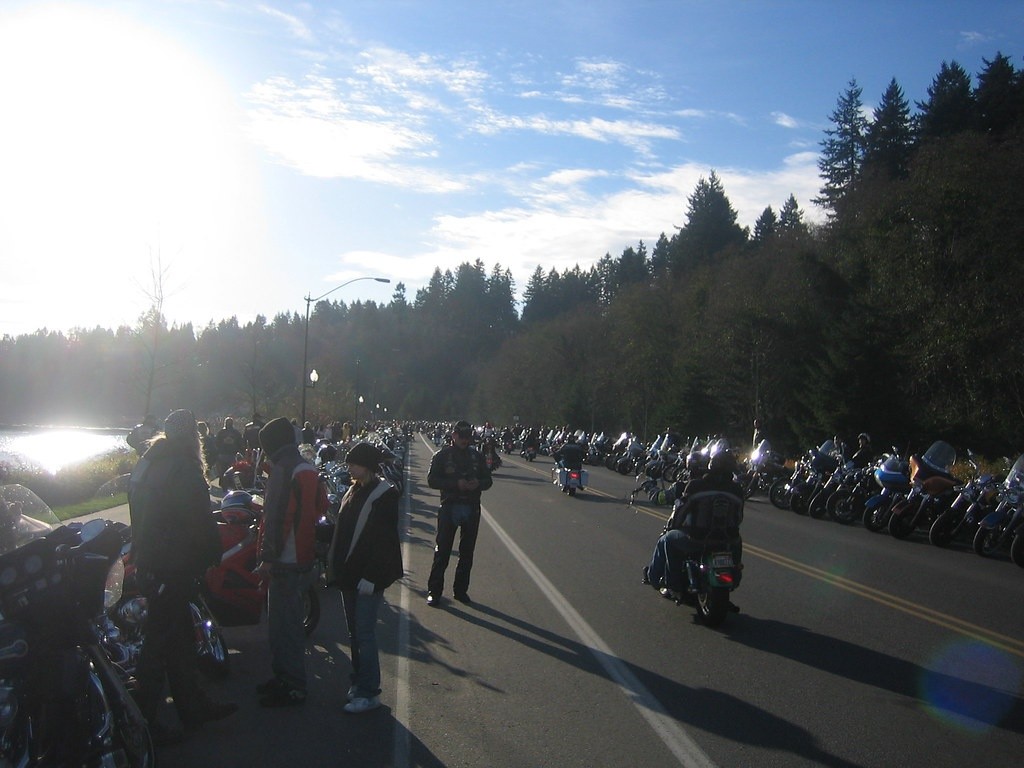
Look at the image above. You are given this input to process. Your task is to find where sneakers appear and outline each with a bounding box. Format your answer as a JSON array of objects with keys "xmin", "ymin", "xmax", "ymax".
[
  {"xmin": 347, "ymin": 686, "xmax": 354, "ymax": 700},
  {"xmin": 185, "ymin": 688, "xmax": 239, "ymax": 723},
  {"xmin": 345, "ymin": 695, "xmax": 381, "ymax": 713}
]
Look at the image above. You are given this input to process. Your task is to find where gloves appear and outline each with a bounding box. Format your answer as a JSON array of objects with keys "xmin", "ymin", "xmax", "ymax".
[{"xmin": 357, "ymin": 578, "xmax": 374, "ymax": 595}]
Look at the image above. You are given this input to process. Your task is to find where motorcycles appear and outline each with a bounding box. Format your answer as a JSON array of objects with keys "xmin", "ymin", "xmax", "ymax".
[
  {"xmin": 1, "ymin": 419, "xmax": 419, "ymax": 768},
  {"xmin": 419, "ymin": 420, "xmax": 1024, "ymax": 568},
  {"xmin": 660, "ymin": 496, "xmax": 741, "ymax": 628},
  {"xmin": 550, "ymin": 446, "xmax": 588, "ymax": 497}
]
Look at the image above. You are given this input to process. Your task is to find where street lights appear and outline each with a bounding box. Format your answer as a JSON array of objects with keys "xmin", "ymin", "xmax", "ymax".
[
  {"xmin": 355, "ymin": 395, "xmax": 388, "ymax": 427},
  {"xmin": 301, "ymin": 277, "xmax": 391, "ymax": 427}
]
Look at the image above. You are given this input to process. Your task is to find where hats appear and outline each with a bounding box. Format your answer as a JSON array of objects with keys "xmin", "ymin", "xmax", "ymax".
[
  {"xmin": 454, "ymin": 419, "xmax": 473, "ymax": 440},
  {"xmin": 345, "ymin": 442, "xmax": 380, "ymax": 472}
]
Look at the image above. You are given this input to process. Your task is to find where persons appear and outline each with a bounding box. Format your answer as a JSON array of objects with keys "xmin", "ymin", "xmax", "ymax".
[
  {"xmin": 291, "ymin": 418, "xmax": 354, "ymax": 446},
  {"xmin": 364, "ymin": 419, "xmax": 584, "ymax": 493},
  {"xmin": 427, "ymin": 421, "xmax": 493, "ymax": 606},
  {"xmin": 128, "ymin": 408, "xmax": 238, "ymax": 725},
  {"xmin": 830, "ymin": 436, "xmax": 849, "ymax": 465},
  {"xmin": 329, "ymin": 442, "xmax": 404, "ymax": 712},
  {"xmin": 648, "ymin": 442, "xmax": 745, "ymax": 594},
  {"xmin": 852, "ymin": 433, "xmax": 874, "ymax": 469},
  {"xmin": 125, "ymin": 412, "xmax": 264, "ymax": 492},
  {"xmin": 753, "ymin": 419, "xmax": 767, "ymax": 447},
  {"xmin": 661, "ymin": 428, "xmax": 671, "ymax": 439},
  {"xmin": 258, "ymin": 416, "xmax": 328, "ymax": 707}
]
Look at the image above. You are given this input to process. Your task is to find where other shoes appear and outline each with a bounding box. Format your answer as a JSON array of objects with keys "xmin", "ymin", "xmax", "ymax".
[
  {"xmin": 259, "ymin": 686, "xmax": 307, "ymax": 706},
  {"xmin": 726, "ymin": 601, "xmax": 740, "ymax": 613},
  {"xmin": 453, "ymin": 592, "xmax": 470, "ymax": 602},
  {"xmin": 255, "ymin": 679, "xmax": 277, "ymax": 693},
  {"xmin": 426, "ymin": 590, "xmax": 440, "ymax": 605},
  {"xmin": 643, "ymin": 566, "xmax": 657, "ymax": 590},
  {"xmin": 659, "ymin": 587, "xmax": 683, "ymax": 601}
]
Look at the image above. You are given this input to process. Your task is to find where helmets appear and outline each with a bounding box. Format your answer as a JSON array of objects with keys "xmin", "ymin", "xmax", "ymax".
[
  {"xmin": 568, "ymin": 435, "xmax": 576, "ymax": 443},
  {"xmin": 221, "ymin": 490, "xmax": 259, "ymax": 524},
  {"xmin": 686, "ymin": 451, "xmax": 708, "ymax": 475},
  {"xmin": 315, "ymin": 438, "xmax": 331, "ymax": 449},
  {"xmin": 316, "ymin": 445, "xmax": 337, "ymax": 462},
  {"xmin": 857, "ymin": 433, "xmax": 870, "ymax": 442},
  {"xmin": 706, "ymin": 451, "xmax": 736, "ymax": 473}
]
